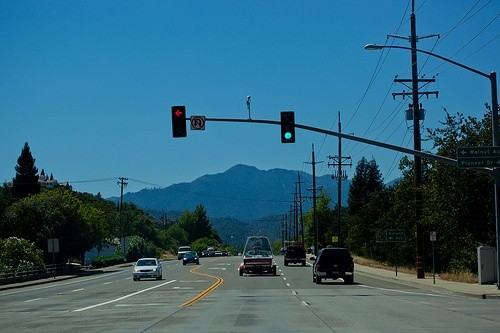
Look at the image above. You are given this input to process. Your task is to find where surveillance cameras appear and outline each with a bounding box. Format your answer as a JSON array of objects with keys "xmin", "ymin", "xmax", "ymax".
[{"xmin": 247, "ymin": 96, "xmax": 251, "ymax": 101}]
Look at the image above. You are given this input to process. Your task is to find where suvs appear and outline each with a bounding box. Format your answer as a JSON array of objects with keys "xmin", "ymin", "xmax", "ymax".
[
  {"xmin": 177, "ymin": 245, "xmax": 190, "ymax": 260},
  {"xmin": 283, "ymin": 246, "xmax": 306, "ymax": 266},
  {"xmin": 309, "ymin": 248, "xmax": 354, "ymax": 285}
]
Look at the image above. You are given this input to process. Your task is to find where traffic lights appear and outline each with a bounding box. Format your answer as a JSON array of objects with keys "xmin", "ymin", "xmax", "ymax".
[
  {"xmin": 281, "ymin": 110, "xmax": 295, "ymax": 144},
  {"xmin": 171, "ymin": 106, "xmax": 187, "ymax": 138}
]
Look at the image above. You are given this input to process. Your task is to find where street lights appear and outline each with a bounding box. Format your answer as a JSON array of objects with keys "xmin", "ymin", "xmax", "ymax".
[{"xmin": 364, "ymin": 43, "xmax": 499, "ymax": 289}]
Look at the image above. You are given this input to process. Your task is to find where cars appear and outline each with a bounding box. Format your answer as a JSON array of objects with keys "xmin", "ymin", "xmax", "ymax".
[
  {"xmin": 182, "ymin": 251, "xmax": 200, "ymax": 264},
  {"xmin": 202, "ymin": 249, "xmax": 229, "ymax": 256},
  {"xmin": 133, "ymin": 257, "xmax": 162, "ymax": 280}
]
